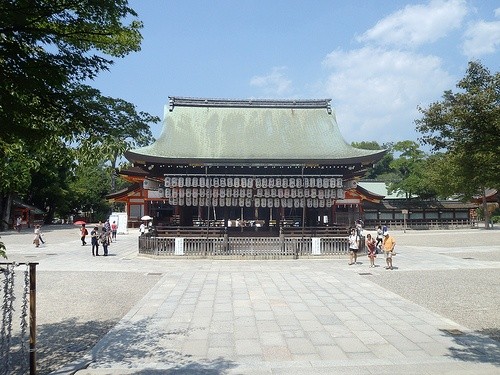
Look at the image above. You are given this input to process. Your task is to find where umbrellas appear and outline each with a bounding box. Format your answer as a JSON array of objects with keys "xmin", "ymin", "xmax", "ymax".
[{"xmin": 73, "ymin": 221, "xmax": 86, "ymax": 230}]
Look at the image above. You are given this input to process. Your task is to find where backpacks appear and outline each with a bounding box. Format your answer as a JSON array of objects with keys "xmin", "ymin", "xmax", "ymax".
[{"xmin": 85, "ymin": 229, "xmax": 88, "ymax": 235}]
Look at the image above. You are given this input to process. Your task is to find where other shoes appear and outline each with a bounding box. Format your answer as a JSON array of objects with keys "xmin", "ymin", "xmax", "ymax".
[
  {"xmin": 96, "ymin": 254, "xmax": 100, "ymax": 256},
  {"xmin": 390, "ymin": 266, "xmax": 393, "ymax": 270},
  {"xmin": 387, "ymin": 266, "xmax": 389, "ymax": 269},
  {"xmin": 373, "ymin": 264, "xmax": 375, "ymax": 268},
  {"xmin": 370, "ymin": 265, "xmax": 372, "ymax": 267},
  {"xmin": 349, "ymin": 262, "xmax": 352, "ymax": 264}
]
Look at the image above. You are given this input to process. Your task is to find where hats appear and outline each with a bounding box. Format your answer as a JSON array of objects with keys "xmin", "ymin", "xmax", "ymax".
[{"xmin": 383, "ymin": 232, "xmax": 389, "ymax": 236}]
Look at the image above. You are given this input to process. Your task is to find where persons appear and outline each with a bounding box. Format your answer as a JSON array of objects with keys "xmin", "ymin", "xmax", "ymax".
[
  {"xmin": 16, "ymin": 216, "xmax": 22, "ymax": 232},
  {"xmin": 148, "ymin": 219, "xmax": 153, "ymax": 232},
  {"xmin": 139, "ymin": 222, "xmax": 145, "ymax": 235},
  {"xmin": 90, "ymin": 220, "xmax": 118, "ymax": 256},
  {"xmin": 365, "ymin": 234, "xmax": 378, "ymax": 268},
  {"xmin": 80, "ymin": 224, "xmax": 87, "ymax": 246},
  {"xmin": 282, "ymin": 218, "xmax": 288, "ymax": 227},
  {"xmin": 381, "ymin": 232, "xmax": 396, "ymax": 270},
  {"xmin": 221, "ymin": 218, "xmax": 261, "ymax": 228},
  {"xmin": 376, "ymin": 224, "xmax": 388, "ymax": 249},
  {"xmin": 347, "ymin": 229, "xmax": 360, "ymax": 265},
  {"xmin": 355, "ymin": 218, "xmax": 364, "ymax": 233},
  {"xmin": 32, "ymin": 224, "xmax": 46, "ymax": 247}
]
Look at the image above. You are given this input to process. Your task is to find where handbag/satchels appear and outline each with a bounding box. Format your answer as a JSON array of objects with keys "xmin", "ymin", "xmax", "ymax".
[
  {"xmin": 378, "ymin": 235, "xmax": 383, "ymax": 239},
  {"xmin": 139, "ymin": 229, "xmax": 141, "ymax": 232},
  {"xmin": 392, "ymin": 249, "xmax": 396, "ymax": 255}
]
